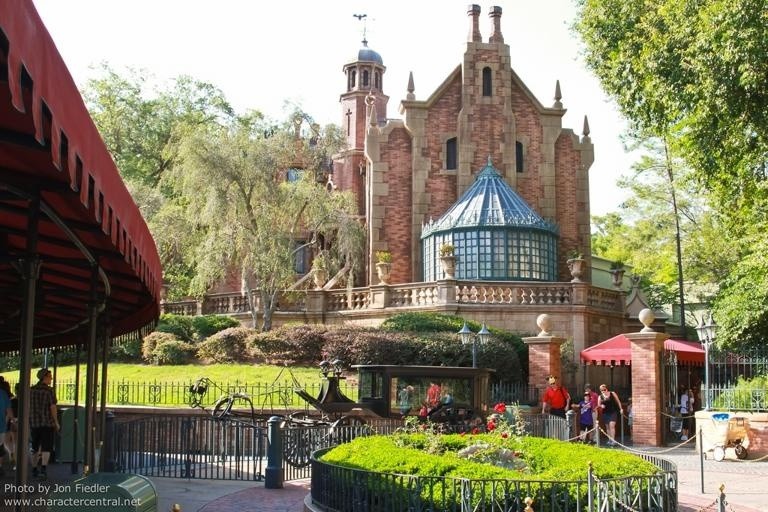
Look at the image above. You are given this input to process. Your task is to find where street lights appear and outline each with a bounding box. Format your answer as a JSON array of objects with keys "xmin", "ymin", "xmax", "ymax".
[
  {"xmin": 457, "ymin": 321, "xmax": 489, "ymax": 366},
  {"xmin": 695, "ymin": 313, "xmax": 721, "ymax": 409},
  {"xmin": 320, "ymin": 360, "xmax": 342, "ymax": 377}
]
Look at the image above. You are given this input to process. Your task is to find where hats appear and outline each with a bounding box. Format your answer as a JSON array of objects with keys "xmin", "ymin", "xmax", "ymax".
[
  {"xmin": 37, "ymin": 369, "xmax": 48, "ymax": 380},
  {"xmin": 584, "ymin": 392, "xmax": 589, "ymax": 394}
]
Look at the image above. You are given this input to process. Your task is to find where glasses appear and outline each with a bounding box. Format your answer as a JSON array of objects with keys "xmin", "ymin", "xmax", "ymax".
[
  {"xmin": 601, "ymin": 389, "xmax": 606, "ymax": 391},
  {"xmin": 551, "ymin": 383, "xmax": 555, "ymax": 385}
]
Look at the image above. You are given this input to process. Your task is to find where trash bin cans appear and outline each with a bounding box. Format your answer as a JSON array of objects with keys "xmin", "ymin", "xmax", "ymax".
[
  {"xmin": 45, "ymin": 473, "xmax": 159, "ymax": 511},
  {"xmin": 57, "ymin": 405, "xmax": 86, "ymax": 461},
  {"xmin": 502, "ymin": 406, "xmax": 530, "ymax": 433}
]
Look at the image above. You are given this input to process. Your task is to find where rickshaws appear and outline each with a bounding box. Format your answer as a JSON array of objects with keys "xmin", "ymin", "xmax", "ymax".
[{"xmin": 698, "ymin": 416, "xmax": 750, "ymax": 461}]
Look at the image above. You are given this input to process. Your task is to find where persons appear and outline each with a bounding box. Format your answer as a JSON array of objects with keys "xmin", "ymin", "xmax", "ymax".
[
  {"xmin": 398, "ymin": 380, "xmax": 454, "ymax": 417},
  {"xmin": 540, "ymin": 374, "xmax": 703, "ymax": 449},
  {"xmin": 0, "ymin": 367, "xmax": 63, "ymax": 481}
]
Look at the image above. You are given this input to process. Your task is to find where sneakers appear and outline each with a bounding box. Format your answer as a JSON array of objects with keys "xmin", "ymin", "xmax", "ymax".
[
  {"xmin": 607, "ymin": 441, "xmax": 615, "ymax": 445},
  {"xmin": 32, "ymin": 470, "xmax": 47, "ymax": 476}
]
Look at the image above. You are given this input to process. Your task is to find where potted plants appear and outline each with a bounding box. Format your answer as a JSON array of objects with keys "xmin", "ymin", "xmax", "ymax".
[
  {"xmin": 374, "ymin": 251, "xmax": 394, "ymax": 286},
  {"xmin": 440, "ymin": 244, "xmax": 456, "ymax": 280},
  {"xmin": 609, "ymin": 259, "xmax": 625, "ymax": 289},
  {"xmin": 310, "ymin": 256, "xmax": 327, "ymax": 289},
  {"xmin": 565, "ymin": 250, "xmax": 588, "ymax": 283}
]
